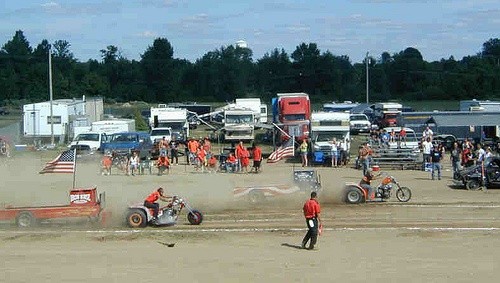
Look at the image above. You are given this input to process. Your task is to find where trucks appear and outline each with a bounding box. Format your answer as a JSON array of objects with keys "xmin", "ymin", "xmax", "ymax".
[
  {"xmin": 92, "ymin": 119, "xmax": 135, "ymax": 141},
  {"xmin": 374, "ymin": 103, "xmax": 402, "ymax": 132},
  {"xmin": 150, "ymin": 105, "xmax": 189, "ymax": 141},
  {"xmin": 22, "ymin": 96, "xmax": 104, "ymax": 144},
  {"xmin": 458, "ymin": 99, "xmax": 500, "ymax": 113},
  {"xmin": 311, "ymin": 125, "xmax": 350, "ymax": 165}
]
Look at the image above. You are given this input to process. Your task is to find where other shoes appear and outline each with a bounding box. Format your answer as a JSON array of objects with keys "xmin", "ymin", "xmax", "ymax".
[
  {"xmin": 302, "ymin": 244, "xmax": 308, "ymax": 249},
  {"xmin": 309, "ymin": 247, "xmax": 313, "ymax": 249},
  {"xmin": 176, "ymin": 163, "xmax": 178, "ymax": 165},
  {"xmin": 170, "ymin": 163, "xmax": 173, "ymax": 165},
  {"xmin": 101, "ymin": 172, "xmax": 104, "ymax": 176},
  {"xmin": 107, "ymin": 172, "xmax": 110, "ymax": 176}
]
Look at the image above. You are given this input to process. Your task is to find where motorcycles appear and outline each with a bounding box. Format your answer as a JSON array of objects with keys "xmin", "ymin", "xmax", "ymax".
[
  {"xmin": 341, "ymin": 172, "xmax": 411, "ymax": 204},
  {"xmin": 125, "ymin": 194, "xmax": 203, "ymax": 228}
]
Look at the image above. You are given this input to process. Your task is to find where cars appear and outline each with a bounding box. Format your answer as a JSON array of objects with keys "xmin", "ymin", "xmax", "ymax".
[{"xmin": 451, "ymin": 157, "xmax": 500, "ymax": 191}]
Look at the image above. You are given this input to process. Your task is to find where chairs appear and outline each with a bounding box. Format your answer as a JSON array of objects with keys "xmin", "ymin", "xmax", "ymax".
[{"xmin": 98, "ymin": 146, "xmax": 263, "ymax": 177}]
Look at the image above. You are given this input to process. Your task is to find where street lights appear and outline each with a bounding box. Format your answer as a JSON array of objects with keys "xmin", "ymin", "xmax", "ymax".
[{"xmin": 366, "ymin": 49, "xmax": 372, "ymax": 105}]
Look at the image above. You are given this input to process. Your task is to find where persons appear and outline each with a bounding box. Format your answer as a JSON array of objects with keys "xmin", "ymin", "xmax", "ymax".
[
  {"xmin": 327, "ymin": 137, "xmax": 348, "ymax": 168},
  {"xmin": 297, "ymin": 139, "xmax": 308, "ymax": 167},
  {"xmin": 422, "ymin": 127, "xmax": 446, "ymax": 181},
  {"xmin": 158, "ymin": 136, "xmax": 182, "ymax": 176},
  {"xmin": 303, "ymin": 192, "xmax": 322, "ymax": 250},
  {"xmin": 127, "ymin": 152, "xmax": 139, "ymax": 176},
  {"xmin": 100, "ymin": 152, "xmax": 113, "ymax": 176},
  {"xmin": 356, "ymin": 142, "xmax": 372, "ymax": 176},
  {"xmin": 450, "ymin": 138, "xmax": 493, "ymax": 171},
  {"xmin": 188, "ymin": 137, "xmax": 219, "ymax": 176},
  {"xmin": 360, "ymin": 168, "xmax": 382, "ymax": 200},
  {"xmin": 226, "ymin": 141, "xmax": 262, "ymax": 175},
  {"xmin": 145, "ymin": 187, "xmax": 173, "ymax": 220},
  {"xmin": 369, "ymin": 126, "xmax": 407, "ymax": 149}
]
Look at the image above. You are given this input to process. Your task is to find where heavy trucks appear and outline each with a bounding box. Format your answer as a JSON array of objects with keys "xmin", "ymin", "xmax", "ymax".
[
  {"xmin": 272, "ymin": 92, "xmax": 312, "ymax": 147},
  {"xmin": 201, "ymin": 105, "xmax": 272, "ymax": 143}
]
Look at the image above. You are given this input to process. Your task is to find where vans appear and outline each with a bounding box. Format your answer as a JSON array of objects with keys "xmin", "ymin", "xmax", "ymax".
[
  {"xmin": 171, "ymin": 128, "xmax": 187, "ymax": 142},
  {"xmin": 105, "ymin": 132, "xmax": 152, "ymax": 155},
  {"xmin": 260, "ymin": 105, "xmax": 268, "ymax": 123},
  {"xmin": 381, "ymin": 127, "xmax": 420, "ymax": 158}
]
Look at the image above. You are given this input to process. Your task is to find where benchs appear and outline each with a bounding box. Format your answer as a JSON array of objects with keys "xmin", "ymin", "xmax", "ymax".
[{"xmin": 354, "ymin": 133, "xmax": 422, "ymax": 169}]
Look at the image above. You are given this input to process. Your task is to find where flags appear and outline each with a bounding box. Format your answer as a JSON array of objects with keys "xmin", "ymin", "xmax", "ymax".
[
  {"xmin": 268, "ymin": 138, "xmax": 295, "ymax": 162},
  {"xmin": 39, "ymin": 149, "xmax": 75, "ymax": 175}
]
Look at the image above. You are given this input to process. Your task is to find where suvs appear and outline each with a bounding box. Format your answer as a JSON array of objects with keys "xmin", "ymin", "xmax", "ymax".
[
  {"xmin": 148, "ymin": 127, "xmax": 172, "ymax": 145},
  {"xmin": 69, "ymin": 132, "xmax": 107, "ymax": 156},
  {"xmin": 348, "ymin": 114, "xmax": 372, "ymax": 134}
]
